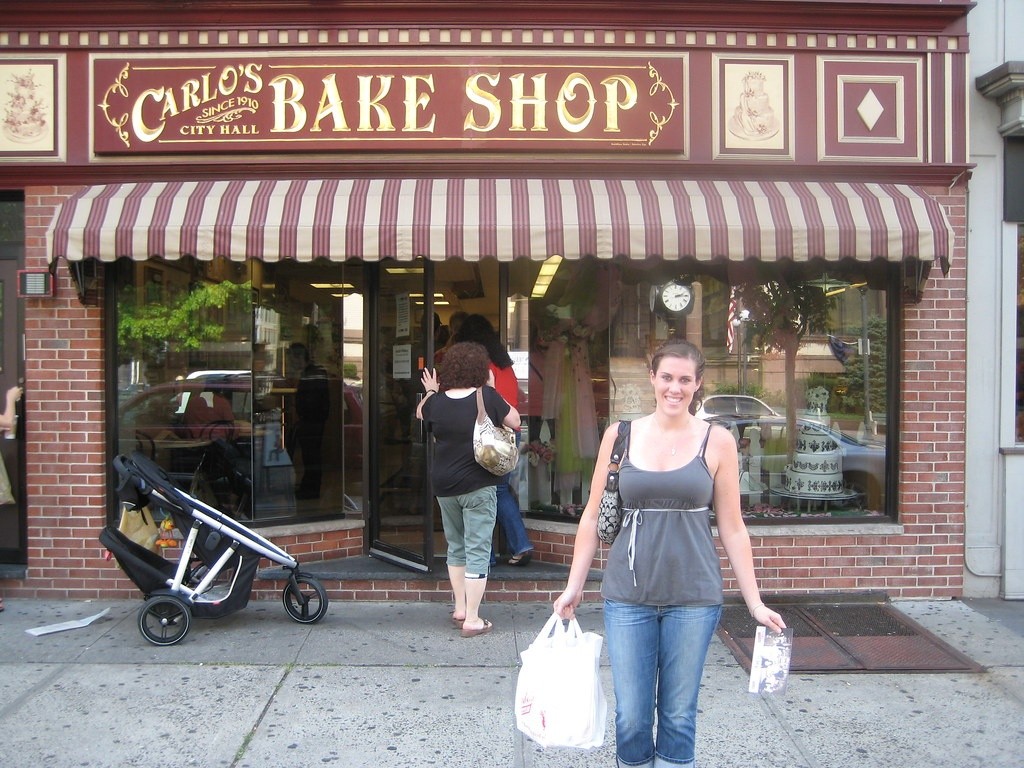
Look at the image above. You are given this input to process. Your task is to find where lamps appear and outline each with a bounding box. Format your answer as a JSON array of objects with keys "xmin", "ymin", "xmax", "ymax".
[
  {"xmin": 899, "ymin": 257, "xmax": 931, "ymax": 303},
  {"xmin": 66, "ymin": 256, "xmax": 102, "ymax": 306}
]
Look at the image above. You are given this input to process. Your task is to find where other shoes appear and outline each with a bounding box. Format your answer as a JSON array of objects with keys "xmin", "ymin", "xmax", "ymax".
[{"xmin": 296, "ymin": 488, "xmax": 319, "ymax": 499}]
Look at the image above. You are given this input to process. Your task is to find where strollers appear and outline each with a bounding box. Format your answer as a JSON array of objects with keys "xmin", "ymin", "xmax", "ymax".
[{"xmin": 93, "ymin": 452, "xmax": 328, "ymax": 645}]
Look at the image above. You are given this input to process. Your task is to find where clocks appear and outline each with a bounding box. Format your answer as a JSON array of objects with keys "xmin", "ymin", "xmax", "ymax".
[{"xmin": 648, "ymin": 278, "xmax": 694, "ymax": 316}]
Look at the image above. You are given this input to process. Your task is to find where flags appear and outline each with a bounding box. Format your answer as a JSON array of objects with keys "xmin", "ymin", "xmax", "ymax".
[{"xmin": 726, "ymin": 286, "xmax": 736, "ymax": 352}]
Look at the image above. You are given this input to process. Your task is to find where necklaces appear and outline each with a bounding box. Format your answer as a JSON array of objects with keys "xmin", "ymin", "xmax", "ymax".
[{"xmin": 652, "ymin": 411, "xmax": 691, "ymax": 455}]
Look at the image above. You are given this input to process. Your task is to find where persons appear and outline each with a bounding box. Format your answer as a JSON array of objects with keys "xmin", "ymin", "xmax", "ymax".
[
  {"xmin": 417, "ymin": 342, "xmax": 520, "ymax": 637},
  {"xmin": 167, "ymin": 360, "xmax": 253, "ymax": 520},
  {"xmin": 388, "ymin": 311, "xmax": 544, "ymax": 567},
  {"xmin": 286, "ymin": 342, "xmax": 330, "ymax": 499},
  {"xmin": 554, "ymin": 340, "xmax": 785, "ymax": 768},
  {"xmin": 0, "ymin": 385, "xmax": 23, "ymax": 612}
]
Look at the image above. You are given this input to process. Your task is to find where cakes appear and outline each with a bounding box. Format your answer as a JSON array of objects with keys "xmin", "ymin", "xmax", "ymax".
[
  {"xmin": 738, "ymin": 453, "xmax": 750, "ymax": 493},
  {"xmin": 784, "ymin": 386, "xmax": 844, "ymax": 495}
]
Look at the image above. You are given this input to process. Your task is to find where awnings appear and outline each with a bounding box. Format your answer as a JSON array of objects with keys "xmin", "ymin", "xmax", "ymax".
[{"xmin": 44, "ymin": 179, "xmax": 956, "ymax": 306}]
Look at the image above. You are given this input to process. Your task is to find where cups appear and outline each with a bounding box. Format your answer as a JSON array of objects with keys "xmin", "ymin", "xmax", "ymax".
[{"xmin": 4, "ymin": 415, "xmax": 19, "ymax": 438}]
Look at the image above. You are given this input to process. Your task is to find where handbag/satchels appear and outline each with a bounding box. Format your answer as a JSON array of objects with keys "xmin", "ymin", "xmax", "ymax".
[
  {"xmin": 596, "ymin": 421, "xmax": 630, "ymax": 545},
  {"xmin": 472, "ymin": 385, "xmax": 520, "ymax": 476},
  {"xmin": 514, "ymin": 612, "xmax": 608, "ymax": 750}
]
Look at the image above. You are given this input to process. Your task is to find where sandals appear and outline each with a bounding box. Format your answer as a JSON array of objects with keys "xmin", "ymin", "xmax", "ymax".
[{"xmin": 509, "ymin": 551, "xmax": 532, "ymax": 567}]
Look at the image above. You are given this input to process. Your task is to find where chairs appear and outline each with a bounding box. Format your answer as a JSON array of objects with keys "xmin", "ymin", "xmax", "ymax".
[
  {"xmin": 192, "ymin": 397, "xmax": 208, "ymax": 420},
  {"xmin": 212, "ymin": 395, "xmax": 233, "ymax": 420}
]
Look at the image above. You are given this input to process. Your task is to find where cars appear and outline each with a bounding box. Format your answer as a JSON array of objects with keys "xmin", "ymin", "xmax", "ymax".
[
  {"xmin": 117, "ymin": 380, "xmax": 363, "ymax": 491},
  {"xmin": 714, "ymin": 418, "xmax": 886, "ymax": 511},
  {"xmin": 695, "ymin": 391, "xmax": 791, "ymax": 432},
  {"xmin": 173, "ymin": 370, "xmax": 252, "ymax": 414}
]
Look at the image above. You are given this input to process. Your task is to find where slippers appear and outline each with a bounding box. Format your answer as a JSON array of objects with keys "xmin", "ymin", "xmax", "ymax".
[
  {"xmin": 461, "ymin": 618, "xmax": 493, "ymax": 638},
  {"xmin": 451, "ymin": 611, "xmax": 465, "ymax": 628}
]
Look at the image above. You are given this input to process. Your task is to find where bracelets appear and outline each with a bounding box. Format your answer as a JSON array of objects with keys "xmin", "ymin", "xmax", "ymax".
[
  {"xmin": 426, "ymin": 389, "xmax": 436, "ymax": 393},
  {"xmin": 751, "ymin": 603, "xmax": 765, "ymax": 617}
]
